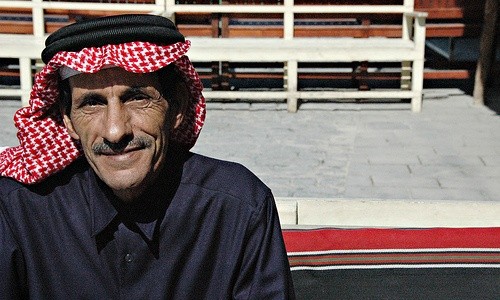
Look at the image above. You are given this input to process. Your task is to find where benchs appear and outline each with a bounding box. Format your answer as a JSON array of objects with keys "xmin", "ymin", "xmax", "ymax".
[
  {"xmin": 0, "ymin": 0, "xmax": 483, "ymax": 113},
  {"xmin": 281, "ymin": 226, "xmax": 500, "ymax": 300}
]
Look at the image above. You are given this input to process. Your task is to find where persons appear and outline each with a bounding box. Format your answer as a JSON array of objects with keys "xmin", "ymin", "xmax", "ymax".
[{"xmin": 0, "ymin": 14, "xmax": 296, "ymax": 300}]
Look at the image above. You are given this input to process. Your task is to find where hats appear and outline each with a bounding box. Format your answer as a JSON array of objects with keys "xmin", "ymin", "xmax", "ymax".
[{"xmin": 0, "ymin": 13, "xmax": 207, "ymax": 187}]
